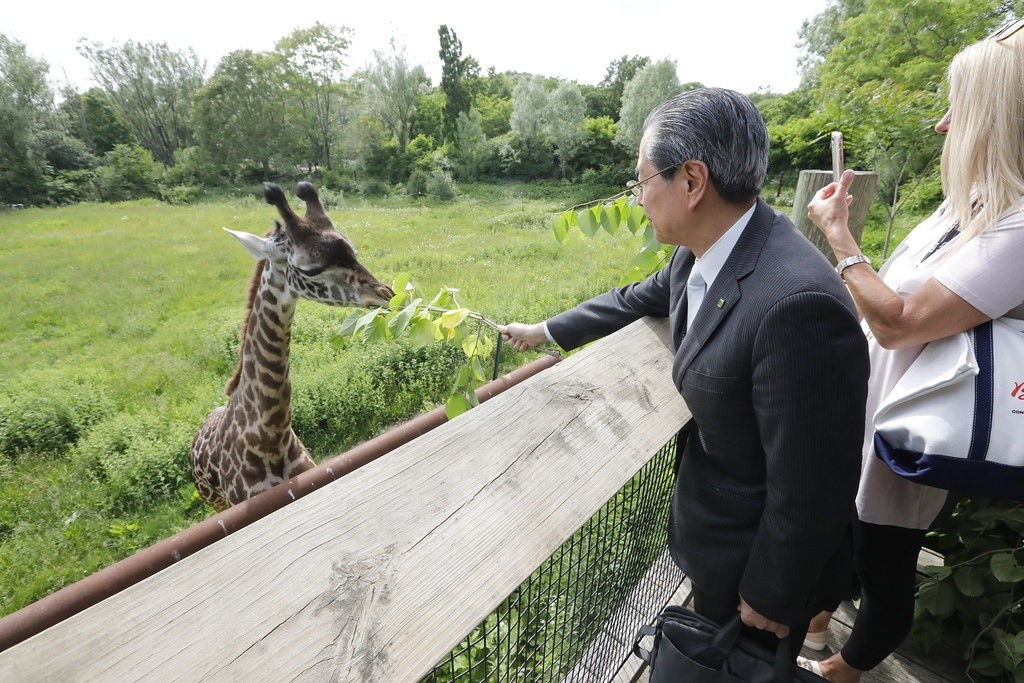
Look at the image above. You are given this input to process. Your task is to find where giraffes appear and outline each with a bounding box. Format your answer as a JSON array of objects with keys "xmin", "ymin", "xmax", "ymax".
[{"xmin": 191, "ymin": 182, "xmax": 399, "ymax": 513}]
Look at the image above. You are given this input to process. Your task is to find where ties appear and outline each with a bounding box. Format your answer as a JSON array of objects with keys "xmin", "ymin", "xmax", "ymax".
[{"xmin": 685, "ymin": 264, "xmax": 707, "ymax": 334}]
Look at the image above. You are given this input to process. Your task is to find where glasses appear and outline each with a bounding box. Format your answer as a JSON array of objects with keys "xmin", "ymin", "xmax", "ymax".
[{"xmin": 626, "ymin": 163, "xmax": 677, "ymax": 197}]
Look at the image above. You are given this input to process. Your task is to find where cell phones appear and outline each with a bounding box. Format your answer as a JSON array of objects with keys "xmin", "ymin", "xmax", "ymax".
[{"xmin": 831, "ymin": 132, "xmax": 844, "ymax": 183}]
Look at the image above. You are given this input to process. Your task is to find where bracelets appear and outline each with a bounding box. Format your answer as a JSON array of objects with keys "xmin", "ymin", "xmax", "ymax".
[{"xmin": 834, "ymin": 256, "xmax": 871, "ymax": 283}]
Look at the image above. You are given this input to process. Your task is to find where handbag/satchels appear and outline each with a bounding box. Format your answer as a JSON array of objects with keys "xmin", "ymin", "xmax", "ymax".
[
  {"xmin": 633, "ymin": 605, "xmax": 831, "ymax": 683},
  {"xmin": 871, "ymin": 314, "xmax": 1024, "ymax": 492}
]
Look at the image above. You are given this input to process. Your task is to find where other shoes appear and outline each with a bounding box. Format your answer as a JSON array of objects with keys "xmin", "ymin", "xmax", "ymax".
[
  {"xmin": 803, "ymin": 630, "xmax": 829, "ymax": 651},
  {"xmin": 796, "ymin": 656, "xmax": 823, "ymax": 677}
]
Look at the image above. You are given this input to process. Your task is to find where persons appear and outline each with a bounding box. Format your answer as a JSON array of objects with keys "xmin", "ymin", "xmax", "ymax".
[
  {"xmin": 797, "ymin": 18, "xmax": 1024, "ymax": 683},
  {"xmin": 498, "ymin": 87, "xmax": 871, "ymax": 660}
]
